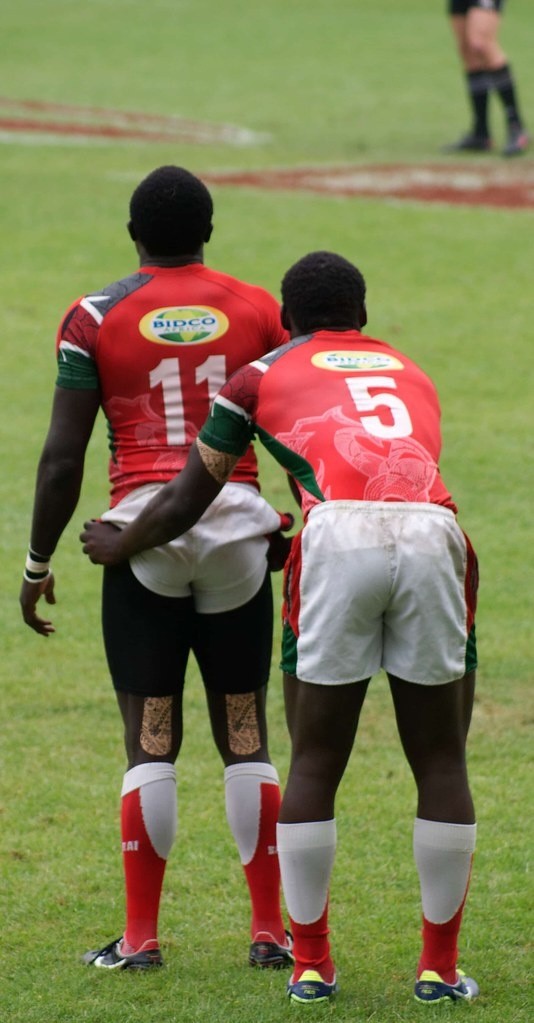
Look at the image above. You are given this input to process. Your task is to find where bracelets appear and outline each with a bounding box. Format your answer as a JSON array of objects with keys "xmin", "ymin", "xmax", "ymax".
[{"xmin": 24, "ymin": 545, "xmax": 52, "ymax": 584}]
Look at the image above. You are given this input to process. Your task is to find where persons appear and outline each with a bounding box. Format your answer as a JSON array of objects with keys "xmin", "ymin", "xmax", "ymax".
[
  {"xmin": 442, "ymin": 0, "xmax": 534, "ymax": 158},
  {"xmin": 79, "ymin": 250, "xmax": 484, "ymax": 1007},
  {"xmin": 17, "ymin": 167, "xmax": 294, "ymax": 978}
]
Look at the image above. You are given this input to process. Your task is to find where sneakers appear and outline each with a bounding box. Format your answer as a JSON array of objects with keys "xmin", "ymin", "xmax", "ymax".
[
  {"xmin": 415, "ymin": 968, "xmax": 480, "ymax": 1005},
  {"xmin": 286, "ymin": 969, "xmax": 337, "ymax": 1004},
  {"xmin": 249, "ymin": 929, "xmax": 294, "ymax": 970},
  {"xmin": 84, "ymin": 935, "xmax": 162, "ymax": 970}
]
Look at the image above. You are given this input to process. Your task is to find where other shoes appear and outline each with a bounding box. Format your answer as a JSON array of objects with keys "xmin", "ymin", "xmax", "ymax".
[
  {"xmin": 505, "ymin": 134, "xmax": 528, "ymax": 156},
  {"xmin": 458, "ymin": 134, "xmax": 491, "ymax": 152}
]
